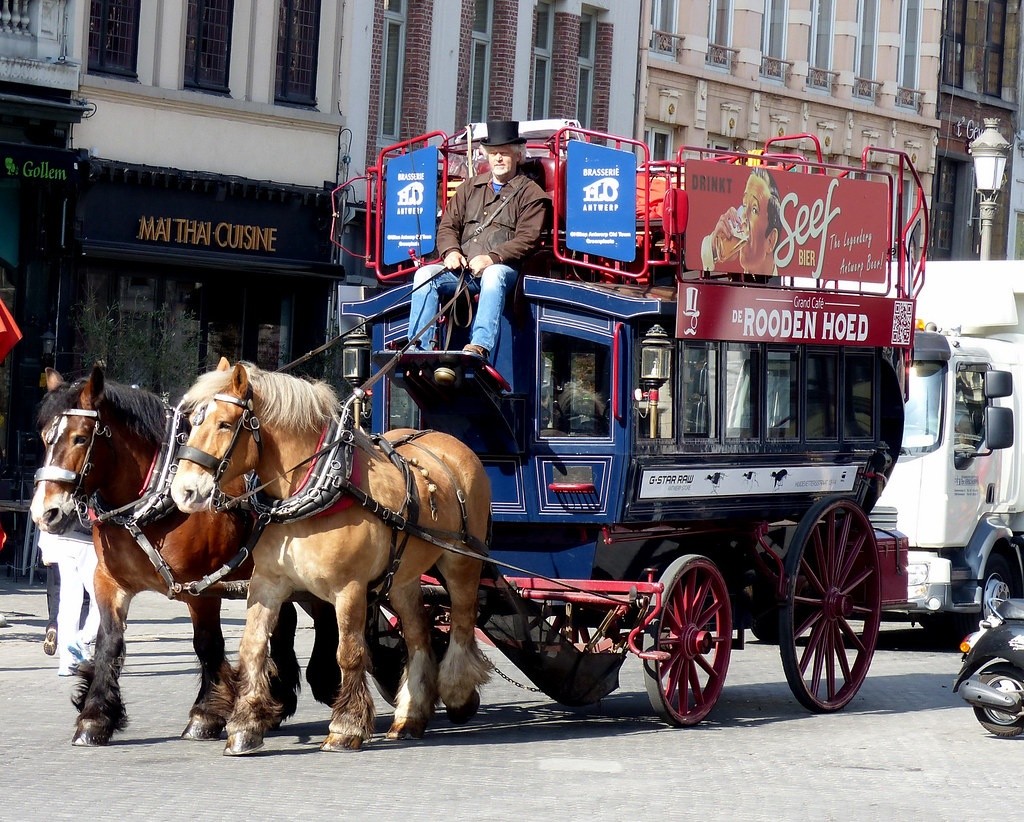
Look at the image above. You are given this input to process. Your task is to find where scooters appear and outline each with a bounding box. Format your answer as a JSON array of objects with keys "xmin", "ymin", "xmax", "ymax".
[{"xmin": 952, "ymin": 572, "xmax": 1024, "ymax": 738}]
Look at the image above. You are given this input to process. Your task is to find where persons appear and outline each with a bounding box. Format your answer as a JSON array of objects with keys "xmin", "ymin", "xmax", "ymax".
[
  {"xmin": 955, "ymin": 414, "xmax": 973, "ymax": 445},
  {"xmin": 404, "ymin": 121, "xmax": 552, "ymax": 361}
]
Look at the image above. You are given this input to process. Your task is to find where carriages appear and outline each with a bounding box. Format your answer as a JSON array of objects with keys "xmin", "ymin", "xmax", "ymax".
[{"xmin": 30, "ymin": 118, "xmax": 930, "ymax": 754}]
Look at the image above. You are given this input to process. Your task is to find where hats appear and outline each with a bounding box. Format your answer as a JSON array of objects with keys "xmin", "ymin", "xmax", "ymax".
[{"xmin": 480, "ymin": 119, "xmax": 527, "ymax": 147}]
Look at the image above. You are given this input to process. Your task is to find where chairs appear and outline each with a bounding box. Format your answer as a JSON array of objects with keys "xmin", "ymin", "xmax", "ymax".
[{"xmin": 470, "ymin": 153, "xmax": 563, "ymax": 317}]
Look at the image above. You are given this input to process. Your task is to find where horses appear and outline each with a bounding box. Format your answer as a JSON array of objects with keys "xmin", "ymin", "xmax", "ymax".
[
  {"xmin": 168, "ymin": 356, "xmax": 490, "ymax": 756},
  {"xmin": 31, "ymin": 363, "xmax": 345, "ymax": 749}
]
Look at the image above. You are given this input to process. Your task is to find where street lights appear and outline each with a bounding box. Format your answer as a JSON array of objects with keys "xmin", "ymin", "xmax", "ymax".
[{"xmin": 970, "ymin": 118, "xmax": 1014, "ymax": 261}]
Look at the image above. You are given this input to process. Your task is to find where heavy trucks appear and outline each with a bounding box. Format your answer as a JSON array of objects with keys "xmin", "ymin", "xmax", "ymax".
[{"xmin": 727, "ymin": 318, "xmax": 1024, "ymax": 648}]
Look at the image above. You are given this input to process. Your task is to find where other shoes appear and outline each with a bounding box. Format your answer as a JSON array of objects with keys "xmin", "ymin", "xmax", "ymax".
[
  {"xmin": 43, "ymin": 628, "xmax": 58, "ymax": 657},
  {"xmin": 405, "ymin": 345, "xmax": 422, "ymax": 352},
  {"xmin": 462, "ymin": 344, "xmax": 490, "ymax": 359}
]
